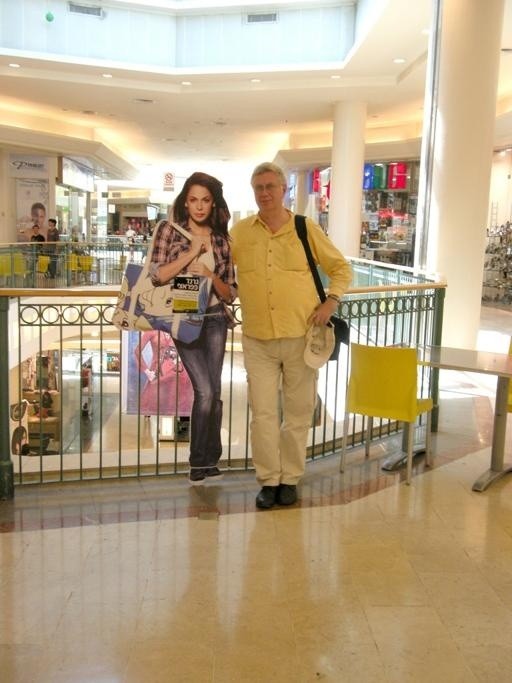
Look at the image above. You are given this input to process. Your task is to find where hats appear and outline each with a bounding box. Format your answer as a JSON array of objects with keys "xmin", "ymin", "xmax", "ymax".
[{"xmin": 304, "ymin": 319, "xmax": 335, "ymax": 368}]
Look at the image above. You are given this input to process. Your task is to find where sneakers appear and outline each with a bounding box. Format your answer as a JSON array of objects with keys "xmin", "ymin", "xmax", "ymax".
[
  {"xmin": 191, "ymin": 467, "xmax": 221, "ymax": 481},
  {"xmin": 256, "ymin": 486, "xmax": 276, "ymax": 508},
  {"xmin": 277, "ymin": 484, "xmax": 296, "ymax": 504}
]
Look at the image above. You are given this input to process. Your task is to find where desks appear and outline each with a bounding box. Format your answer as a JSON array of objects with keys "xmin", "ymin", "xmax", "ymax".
[
  {"xmin": 28, "ymin": 416, "xmax": 60, "ymax": 440},
  {"xmin": 381, "ymin": 344, "xmax": 511, "ymax": 492}
]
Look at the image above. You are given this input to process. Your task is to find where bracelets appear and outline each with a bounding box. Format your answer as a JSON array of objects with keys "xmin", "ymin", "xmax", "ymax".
[
  {"xmin": 328, "ymin": 294, "xmax": 340, "ymax": 301},
  {"xmin": 211, "ymin": 272, "xmax": 217, "ymax": 280}
]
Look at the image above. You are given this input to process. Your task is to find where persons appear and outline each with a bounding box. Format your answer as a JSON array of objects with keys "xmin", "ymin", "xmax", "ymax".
[
  {"xmin": 46, "ymin": 220, "xmax": 61, "ymax": 276},
  {"xmin": 229, "ymin": 164, "xmax": 354, "ymax": 511},
  {"xmin": 29, "ymin": 226, "xmax": 45, "ymax": 248},
  {"xmin": 134, "ymin": 330, "xmax": 197, "ymax": 418},
  {"xmin": 43, "ymin": 391, "xmax": 52, "ymax": 414},
  {"xmin": 147, "ymin": 172, "xmax": 238, "ymax": 487},
  {"xmin": 22, "ymin": 203, "xmax": 48, "ymax": 241}
]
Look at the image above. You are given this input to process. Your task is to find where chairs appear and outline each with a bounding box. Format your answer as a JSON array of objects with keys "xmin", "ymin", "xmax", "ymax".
[
  {"xmin": 109, "ymin": 256, "xmax": 127, "ymax": 285},
  {"xmin": 337, "ymin": 340, "xmax": 435, "ymax": 485},
  {"xmin": 1, "ymin": 252, "xmax": 99, "ymax": 288}
]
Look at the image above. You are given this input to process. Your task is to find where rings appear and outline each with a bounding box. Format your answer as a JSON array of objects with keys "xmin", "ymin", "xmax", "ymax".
[{"xmin": 313, "ymin": 313, "xmax": 317, "ymax": 319}]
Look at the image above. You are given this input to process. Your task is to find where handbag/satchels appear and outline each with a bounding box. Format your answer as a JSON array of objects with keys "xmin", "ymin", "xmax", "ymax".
[
  {"xmin": 112, "ymin": 260, "xmax": 214, "ymax": 344},
  {"xmin": 329, "ymin": 316, "xmax": 349, "ymax": 359}
]
